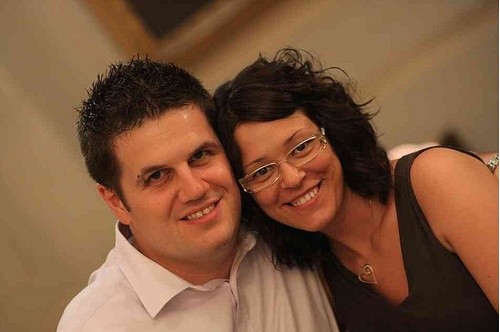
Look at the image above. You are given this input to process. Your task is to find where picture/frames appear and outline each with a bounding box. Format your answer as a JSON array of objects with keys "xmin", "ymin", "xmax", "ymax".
[{"xmin": 84, "ymin": 0, "xmax": 270, "ymax": 74}]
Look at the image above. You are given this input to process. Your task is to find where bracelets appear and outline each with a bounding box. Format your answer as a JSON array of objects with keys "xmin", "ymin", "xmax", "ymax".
[{"xmin": 487, "ymin": 152, "xmax": 498, "ymax": 173}]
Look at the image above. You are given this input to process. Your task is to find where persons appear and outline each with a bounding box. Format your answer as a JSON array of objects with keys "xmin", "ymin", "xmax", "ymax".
[
  {"xmin": 209, "ymin": 45, "xmax": 499, "ymax": 332},
  {"xmin": 55, "ymin": 51, "xmax": 499, "ymax": 332}
]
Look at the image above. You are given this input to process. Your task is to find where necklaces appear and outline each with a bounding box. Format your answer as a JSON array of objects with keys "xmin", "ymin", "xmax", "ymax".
[{"xmin": 333, "ymin": 198, "xmax": 379, "ymax": 285}]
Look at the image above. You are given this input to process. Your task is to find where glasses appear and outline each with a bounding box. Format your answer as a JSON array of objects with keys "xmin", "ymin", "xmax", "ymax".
[{"xmin": 239, "ymin": 127, "xmax": 326, "ymax": 194}]
